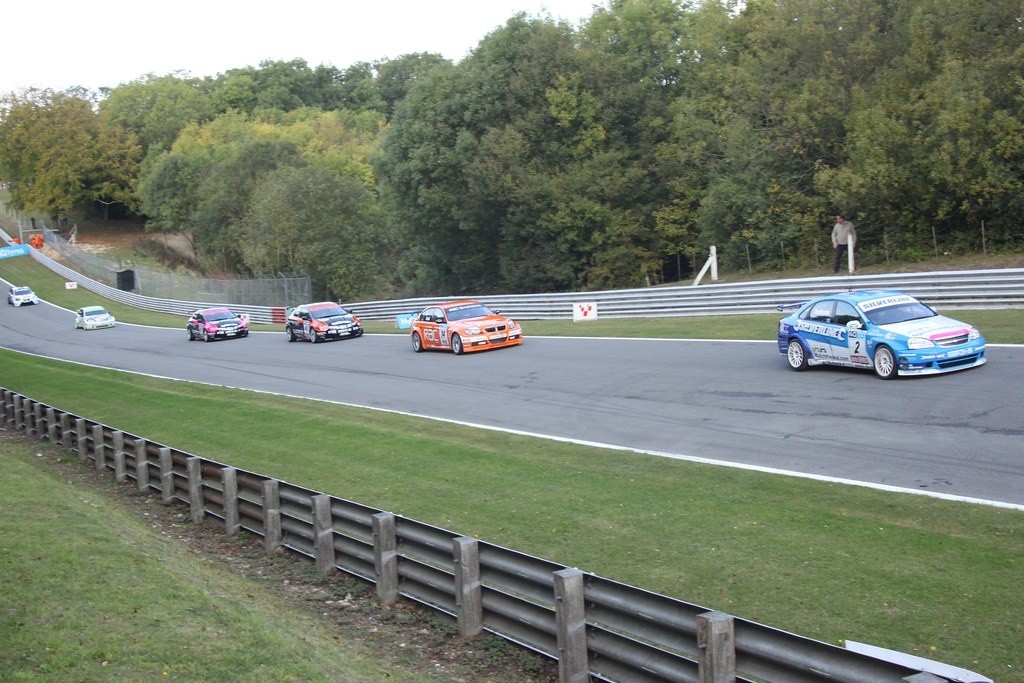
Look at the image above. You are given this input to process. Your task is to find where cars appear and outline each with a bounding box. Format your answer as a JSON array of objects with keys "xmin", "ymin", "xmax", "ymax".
[
  {"xmin": 284, "ymin": 300, "xmax": 364, "ymax": 344},
  {"xmin": 74, "ymin": 306, "xmax": 116, "ymax": 332},
  {"xmin": 776, "ymin": 291, "xmax": 989, "ymax": 379},
  {"xmin": 409, "ymin": 300, "xmax": 523, "ymax": 355},
  {"xmin": 7, "ymin": 286, "xmax": 39, "ymax": 308},
  {"xmin": 185, "ymin": 306, "xmax": 249, "ymax": 343}
]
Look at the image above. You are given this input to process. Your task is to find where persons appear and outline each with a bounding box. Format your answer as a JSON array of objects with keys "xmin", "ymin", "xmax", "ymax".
[
  {"xmin": 831, "ymin": 214, "xmax": 857, "ymax": 274},
  {"xmin": 8, "ymin": 232, "xmax": 44, "ymax": 250}
]
